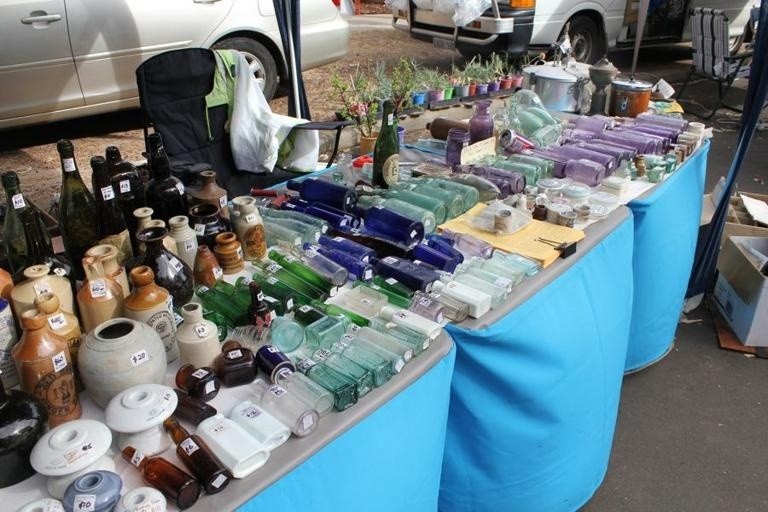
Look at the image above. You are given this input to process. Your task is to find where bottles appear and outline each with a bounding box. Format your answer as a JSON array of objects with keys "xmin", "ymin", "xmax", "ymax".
[{"xmin": 0, "ymin": 97, "xmax": 709, "ymax": 511}]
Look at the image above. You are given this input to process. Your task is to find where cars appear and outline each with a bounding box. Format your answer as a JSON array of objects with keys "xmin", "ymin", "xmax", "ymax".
[{"xmin": 0, "ymin": 1, "xmax": 361, "ymax": 138}]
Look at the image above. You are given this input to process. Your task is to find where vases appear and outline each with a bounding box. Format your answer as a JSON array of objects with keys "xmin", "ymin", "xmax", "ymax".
[{"xmin": 0, "ymin": 171, "xmax": 269, "ymax": 510}]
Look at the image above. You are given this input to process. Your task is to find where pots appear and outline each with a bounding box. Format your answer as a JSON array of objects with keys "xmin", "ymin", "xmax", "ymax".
[{"xmin": 518, "ymin": 52, "xmax": 650, "ymax": 119}]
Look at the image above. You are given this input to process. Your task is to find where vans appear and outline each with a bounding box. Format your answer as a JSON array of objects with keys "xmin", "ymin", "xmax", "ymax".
[{"xmin": 386, "ymin": 0, "xmax": 761, "ymax": 67}]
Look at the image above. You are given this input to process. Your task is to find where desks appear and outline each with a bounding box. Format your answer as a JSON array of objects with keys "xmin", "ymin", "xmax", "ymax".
[
  {"xmin": 252, "ymin": 143, "xmax": 632, "ymax": 512},
  {"xmin": 2, "ymin": 219, "xmax": 453, "ymax": 511},
  {"xmin": 460, "ymin": 86, "xmax": 711, "ymax": 373}
]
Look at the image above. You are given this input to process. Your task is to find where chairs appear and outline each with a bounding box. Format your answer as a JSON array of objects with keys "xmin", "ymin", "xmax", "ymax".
[
  {"xmin": 133, "ymin": 50, "xmax": 354, "ymax": 196},
  {"xmin": 679, "ymin": 7, "xmax": 768, "ymax": 119}
]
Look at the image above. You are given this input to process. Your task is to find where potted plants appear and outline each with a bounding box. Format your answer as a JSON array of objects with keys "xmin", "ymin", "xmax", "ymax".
[{"xmin": 330, "ymin": 49, "xmax": 548, "ymax": 156}]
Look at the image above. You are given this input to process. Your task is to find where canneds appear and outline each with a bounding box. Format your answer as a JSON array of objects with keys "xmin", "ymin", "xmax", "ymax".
[{"xmin": 497, "ymin": 130, "xmax": 535, "ymax": 154}]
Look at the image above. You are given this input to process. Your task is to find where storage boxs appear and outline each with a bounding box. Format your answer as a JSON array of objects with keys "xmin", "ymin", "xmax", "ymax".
[{"xmin": 714, "ymin": 188, "xmax": 768, "ymax": 350}]
